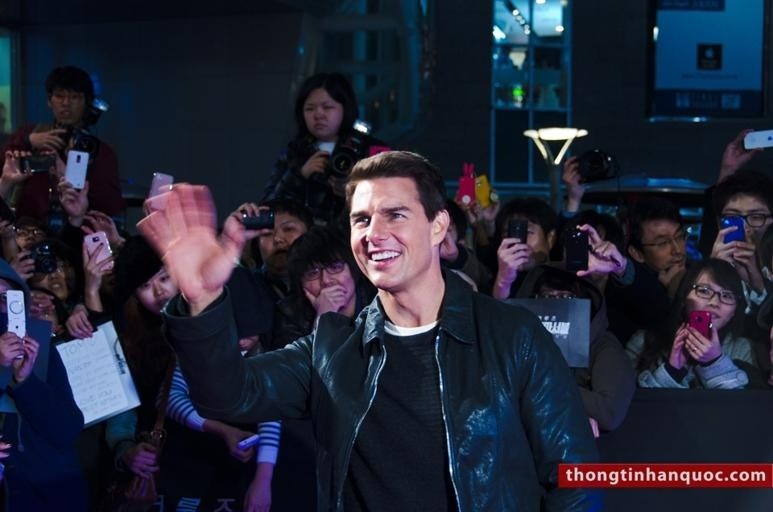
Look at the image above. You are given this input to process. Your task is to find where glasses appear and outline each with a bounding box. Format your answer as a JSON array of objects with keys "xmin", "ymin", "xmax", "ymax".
[
  {"xmin": 693, "ymin": 284, "xmax": 736, "ymax": 307},
  {"xmin": 301, "ymin": 262, "xmax": 348, "ymax": 283},
  {"xmin": 725, "ymin": 210, "xmax": 771, "ymax": 227},
  {"xmin": 637, "ymin": 231, "xmax": 692, "ymax": 248},
  {"xmin": 49, "ymin": 90, "xmax": 83, "ymax": 106},
  {"xmin": 534, "ymin": 291, "xmax": 579, "ymax": 301}
]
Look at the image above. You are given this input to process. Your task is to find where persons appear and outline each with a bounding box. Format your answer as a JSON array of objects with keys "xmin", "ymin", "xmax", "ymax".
[{"xmin": 0, "ymin": 67, "xmax": 773, "ymax": 512}]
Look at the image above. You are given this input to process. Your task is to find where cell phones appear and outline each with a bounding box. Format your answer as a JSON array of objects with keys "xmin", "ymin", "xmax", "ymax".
[
  {"xmin": 244, "ymin": 211, "xmax": 274, "ymax": 230},
  {"xmin": 744, "ymin": 130, "xmax": 773, "ymax": 149},
  {"xmin": 238, "ymin": 434, "xmax": 260, "ymax": 451},
  {"xmin": 565, "ymin": 226, "xmax": 589, "ymax": 271},
  {"xmin": 6, "ymin": 290, "xmax": 26, "ymax": 359},
  {"xmin": 507, "ymin": 218, "xmax": 528, "ymax": 248},
  {"xmin": 690, "ymin": 311, "xmax": 712, "ymax": 341},
  {"xmin": 148, "ymin": 173, "xmax": 174, "ymax": 198},
  {"xmin": 84, "ymin": 231, "xmax": 114, "ymax": 271},
  {"xmin": 20, "ymin": 156, "xmax": 54, "ymax": 172},
  {"xmin": 720, "ymin": 215, "xmax": 746, "ymax": 244},
  {"xmin": 64, "ymin": 150, "xmax": 89, "ymax": 190}
]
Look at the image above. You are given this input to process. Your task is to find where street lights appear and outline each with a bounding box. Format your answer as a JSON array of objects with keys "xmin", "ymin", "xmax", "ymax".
[{"xmin": 519, "ymin": 125, "xmax": 592, "ymax": 214}]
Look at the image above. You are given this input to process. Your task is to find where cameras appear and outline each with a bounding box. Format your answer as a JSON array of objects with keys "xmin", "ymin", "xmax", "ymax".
[
  {"xmin": 572, "ymin": 149, "xmax": 620, "ymax": 184},
  {"xmin": 59, "ymin": 124, "xmax": 99, "ymax": 157},
  {"xmin": 326, "ymin": 119, "xmax": 372, "ymax": 178},
  {"xmin": 23, "ymin": 241, "xmax": 57, "ymax": 273}
]
